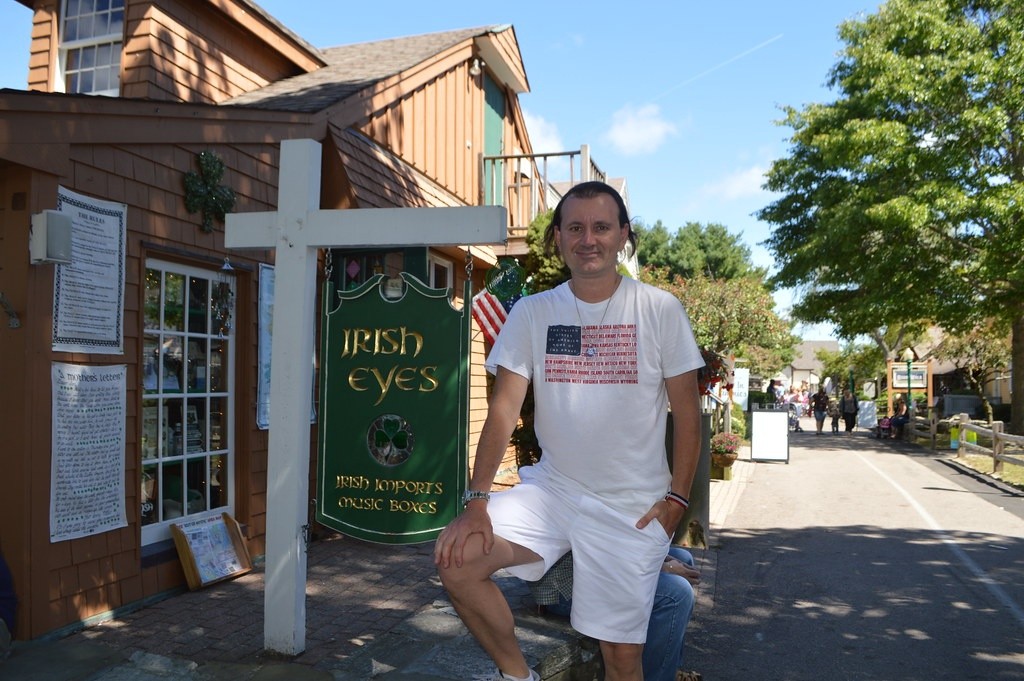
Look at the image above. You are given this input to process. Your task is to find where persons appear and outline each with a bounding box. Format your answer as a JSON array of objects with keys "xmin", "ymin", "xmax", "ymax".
[
  {"xmin": 527, "ymin": 548, "xmax": 701, "ymax": 681},
  {"xmin": 435, "ymin": 182, "xmax": 705, "ymax": 681},
  {"xmin": 767, "ymin": 379, "xmax": 859, "ymax": 436},
  {"xmin": 879, "ymin": 392, "xmax": 910, "ymax": 439}
]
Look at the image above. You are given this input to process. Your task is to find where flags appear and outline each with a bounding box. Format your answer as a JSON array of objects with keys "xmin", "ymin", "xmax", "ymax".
[{"xmin": 472, "ymin": 273, "xmax": 524, "ymax": 345}]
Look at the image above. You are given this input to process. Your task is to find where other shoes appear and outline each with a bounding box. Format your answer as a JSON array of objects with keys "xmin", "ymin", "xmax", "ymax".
[
  {"xmin": 471, "ymin": 666, "xmax": 540, "ymax": 681},
  {"xmin": 675, "ymin": 669, "xmax": 703, "ymax": 681}
]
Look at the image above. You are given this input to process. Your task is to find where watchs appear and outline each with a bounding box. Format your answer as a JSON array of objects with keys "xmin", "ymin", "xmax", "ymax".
[{"xmin": 462, "ymin": 490, "xmax": 490, "ymax": 504}]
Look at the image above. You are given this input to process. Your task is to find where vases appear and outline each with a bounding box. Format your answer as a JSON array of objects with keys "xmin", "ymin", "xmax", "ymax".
[{"xmin": 712, "ymin": 453, "xmax": 738, "ymax": 468}]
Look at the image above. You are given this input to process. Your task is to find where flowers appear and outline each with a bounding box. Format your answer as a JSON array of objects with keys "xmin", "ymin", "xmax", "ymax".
[
  {"xmin": 698, "ymin": 344, "xmax": 735, "ymax": 396},
  {"xmin": 711, "ymin": 432, "xmax": 742, "ymax": 455}
]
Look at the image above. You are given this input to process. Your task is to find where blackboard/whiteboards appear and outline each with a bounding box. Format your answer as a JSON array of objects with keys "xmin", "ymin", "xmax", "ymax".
[{"xmin": 751, "ymin": 409, "xmax": 789, "ymax": 462}]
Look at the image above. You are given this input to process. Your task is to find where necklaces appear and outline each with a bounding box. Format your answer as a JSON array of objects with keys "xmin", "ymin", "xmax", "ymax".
[{"xmin": 572, "ymin": 276, "xmax": 618, "ymax": 357}]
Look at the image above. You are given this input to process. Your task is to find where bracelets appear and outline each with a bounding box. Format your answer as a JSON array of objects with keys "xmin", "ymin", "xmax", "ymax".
[{"xmin": 665, "ymin": 491, "xmax": 690, "ymax": 510}]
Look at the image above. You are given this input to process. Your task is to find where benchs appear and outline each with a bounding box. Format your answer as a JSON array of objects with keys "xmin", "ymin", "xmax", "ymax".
[{"xmin": 895, "ymin": 423, "xmax": 909, "ymax": 440}]
